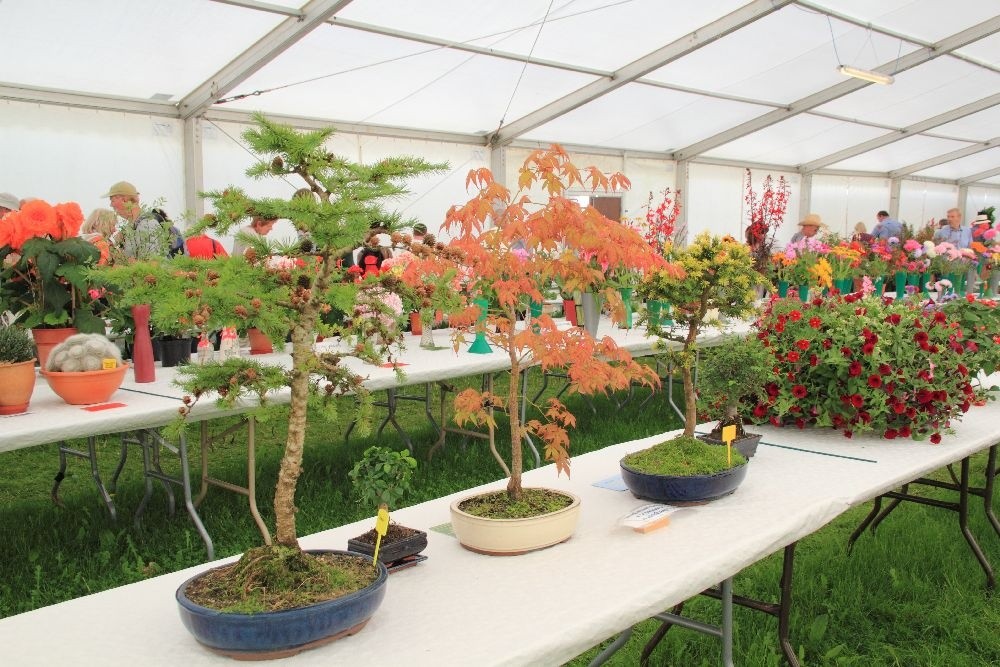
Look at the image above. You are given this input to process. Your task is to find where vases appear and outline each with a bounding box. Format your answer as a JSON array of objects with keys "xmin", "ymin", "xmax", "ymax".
[{"xmin": 30, "ymin": 328, "xmax": 78, "ymax": 369}]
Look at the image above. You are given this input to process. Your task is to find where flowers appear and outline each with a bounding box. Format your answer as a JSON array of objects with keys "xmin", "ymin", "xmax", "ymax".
[
  {"xmin": 377, "ymin": 252, "xmax": 412, "ymax": 277},
  {"xmin": 345, "ymin": 287, "xmax": 402, "ymax": 328},
  {"xmin": 0, "ymin": 198, "xmax": 107, "ymax": 338},
  {"xmin": 578, "ymin": 167, "xmax": 1000, "ymax": 445}
]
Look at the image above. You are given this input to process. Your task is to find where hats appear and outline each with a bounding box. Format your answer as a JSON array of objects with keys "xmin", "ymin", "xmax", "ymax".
[
  {"xmin": 797, "ymin": 214, "xmax": 827, "ymax": 228},
  {"xmin": 101, "ymin": 182, "xmax": 139, "ymax": 198},
  {"xmin": 973, "ymin": 214, "xmax": 991, "ymax": 225}
]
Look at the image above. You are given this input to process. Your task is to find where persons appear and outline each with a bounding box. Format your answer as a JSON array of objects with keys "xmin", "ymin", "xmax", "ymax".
[
  {"xmin": 743, "ymin": 208, "xmax": 1000, "ymax": 298},
  {"xmin": 0, "ymin": 181, "xmax": 437, "ymax": 285}
]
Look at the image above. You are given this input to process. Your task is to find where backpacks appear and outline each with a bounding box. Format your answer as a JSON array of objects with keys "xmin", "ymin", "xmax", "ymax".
[{"xmin": 134, "ymin": 209, "xmax": 183, "ymax": 249}]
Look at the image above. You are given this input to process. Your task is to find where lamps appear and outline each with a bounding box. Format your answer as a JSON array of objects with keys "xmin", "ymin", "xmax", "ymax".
[{"xmin": 826, "ymin": 14, "xmax": 903, "ymax": 85}]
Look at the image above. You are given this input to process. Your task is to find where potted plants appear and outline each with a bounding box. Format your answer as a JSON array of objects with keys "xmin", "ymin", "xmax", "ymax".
[
  {"xmin": 39, "ymin": 332, "xmax": 133, "ymax": 405},
  {"xmin": 0, "ymin": 325, "xmax": 37, "ymax": 414},
  {"xmin": 83, "ymin": 109, "xmax": 451, "ymax": 662},
  {"xmin": 618, "ymin": 231, "xmax": 777, "ymax": 506},
  {"xmin": 439, "ymin": 142, "xmax": 687, "ymax": 558},
  {"xmin": 694, "ymin": 334, "xmax": 777, "ymax": 457}
]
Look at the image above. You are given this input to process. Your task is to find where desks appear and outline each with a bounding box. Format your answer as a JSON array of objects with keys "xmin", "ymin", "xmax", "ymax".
[{"xmin": 0, "ymin": 301, "xmax": 1000, "ymax": 667}]
